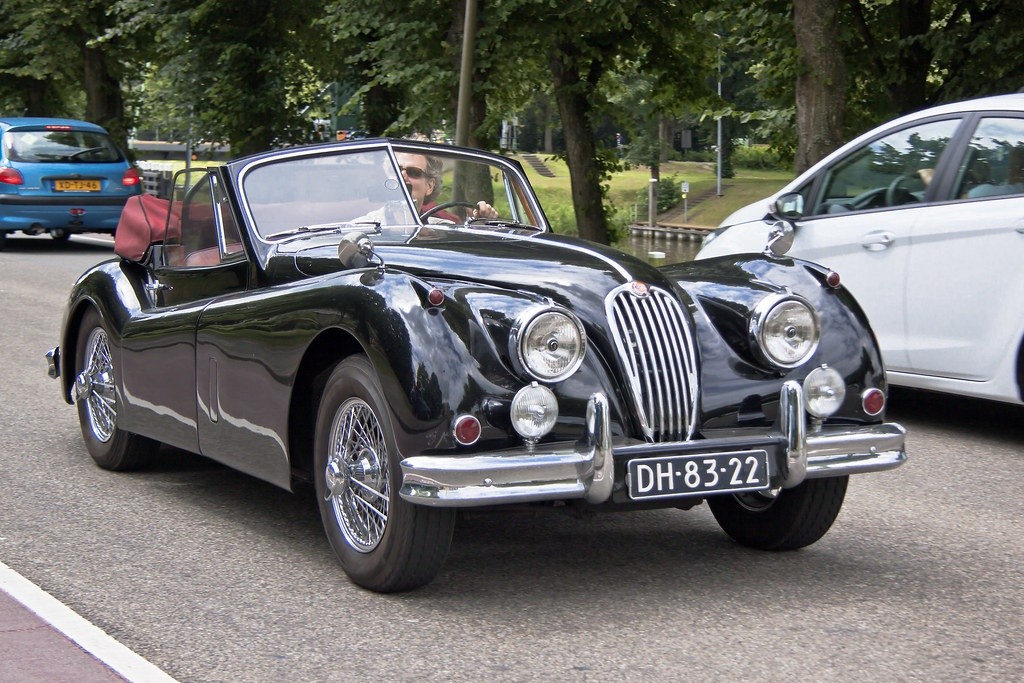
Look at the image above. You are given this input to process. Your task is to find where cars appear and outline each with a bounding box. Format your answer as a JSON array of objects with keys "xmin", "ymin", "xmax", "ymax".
[
  {"xmin": 49, "ymin": 139, "xmax": 907, "ymax": 594},
  {"xmin": 0, "ymin": 118, "xmax": 139, "ymax": 243},
  {"xmin": 693, "ymin": 93, "xmax": 1024, "ymax": 406}
]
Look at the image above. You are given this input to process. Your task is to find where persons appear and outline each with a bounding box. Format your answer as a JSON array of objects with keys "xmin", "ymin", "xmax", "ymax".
[
  {"xmin": 916, "ymin": 146, "xmax": 1024, "ymax": 199},
  {"xmin": 349, "ymin": 132, "xmax": 499, "ymax": 227}
]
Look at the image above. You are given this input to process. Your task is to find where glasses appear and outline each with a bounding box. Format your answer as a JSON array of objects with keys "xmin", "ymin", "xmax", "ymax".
[{"xmin": 389, "ymin": 164, "xmax": 430, "ymax": 180}]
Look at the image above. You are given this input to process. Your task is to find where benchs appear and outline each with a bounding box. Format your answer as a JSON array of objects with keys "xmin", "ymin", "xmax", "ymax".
[{"xmin": 185, "ymin": 242, "xmax": 242, "ymax": 267}]
